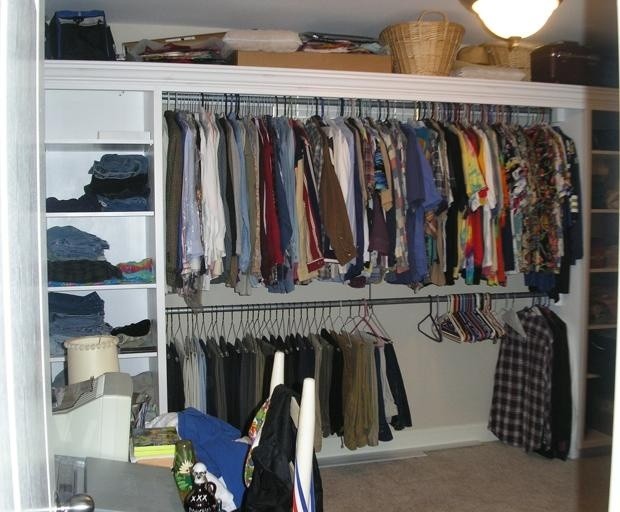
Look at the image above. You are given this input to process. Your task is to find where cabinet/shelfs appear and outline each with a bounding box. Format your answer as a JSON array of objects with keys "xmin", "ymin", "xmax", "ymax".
[
  {"xmin": 37, "ymin": 59, "xmax": 169, "ymax": 512},
  {"xmin": 569, "ymin": 82, "xmax": 619, "ymax": 461}
]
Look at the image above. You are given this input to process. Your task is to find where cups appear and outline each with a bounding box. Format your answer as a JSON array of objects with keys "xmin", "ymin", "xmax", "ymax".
[{"xmin": 58, "ymin": 456, "xmax": 86, "ymax": 504}]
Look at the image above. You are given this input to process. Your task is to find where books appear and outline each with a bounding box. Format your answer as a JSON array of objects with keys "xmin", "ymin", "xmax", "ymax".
[{"xmin": 132, "ymin": 426, "xmax": 178, "ymax": 458}]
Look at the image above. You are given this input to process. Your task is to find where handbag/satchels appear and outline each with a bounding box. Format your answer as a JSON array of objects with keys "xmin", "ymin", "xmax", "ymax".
[{"xmin": 45, "ymin": 9, "xmax": 117, "ymax": 61}]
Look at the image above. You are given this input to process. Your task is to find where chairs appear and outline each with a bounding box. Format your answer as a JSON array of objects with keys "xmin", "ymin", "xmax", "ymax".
[{"xmin": 269, "ymin": 352, "xmax": 316, "ymax": 510}]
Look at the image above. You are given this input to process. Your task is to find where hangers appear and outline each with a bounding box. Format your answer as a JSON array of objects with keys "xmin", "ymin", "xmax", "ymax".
[
  {"xmin": 527, "ymin": 289, "xmax": 551, "ymax": 314},
  {"xmin": 418, "ymin": 293, "xmax": 528, "ymax": 344},
  {"xmin": 164, "ymin": 298, "xmax": 395, "ymax": 358},
  {"xmin": 162, "ymin": 92, "xmax": 554, "ymax": 128}
]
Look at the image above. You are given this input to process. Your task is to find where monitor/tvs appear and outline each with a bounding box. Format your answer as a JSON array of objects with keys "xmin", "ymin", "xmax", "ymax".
[{"xmin": 51, "ymin": 372, "xmax": 133, "ymax": 462}]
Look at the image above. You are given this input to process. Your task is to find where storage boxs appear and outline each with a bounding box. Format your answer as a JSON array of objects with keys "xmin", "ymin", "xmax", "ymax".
[{"xmin": 531, "ymin": 41, "xmax": 618, "ymax": 87}]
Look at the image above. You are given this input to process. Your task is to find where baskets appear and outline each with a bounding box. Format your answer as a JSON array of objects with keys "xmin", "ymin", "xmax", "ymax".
[
  {"xmin": 379, "ymin": 10, "xmax": 465, "ymax": 83},
  {"xmin": 458, "ymin": 36, "xmax": 542, "ymax": 83}
]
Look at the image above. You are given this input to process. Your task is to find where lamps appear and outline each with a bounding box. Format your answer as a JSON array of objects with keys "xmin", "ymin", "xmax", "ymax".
[{"xmin": 469, "ymin": 0, "xmax": 563, "ymax": 49}]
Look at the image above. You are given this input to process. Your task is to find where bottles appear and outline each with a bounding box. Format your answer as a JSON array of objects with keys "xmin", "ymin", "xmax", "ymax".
[{"xmin": 174, "ymin": 438, "xmax": 221, "ymax": 512}]
